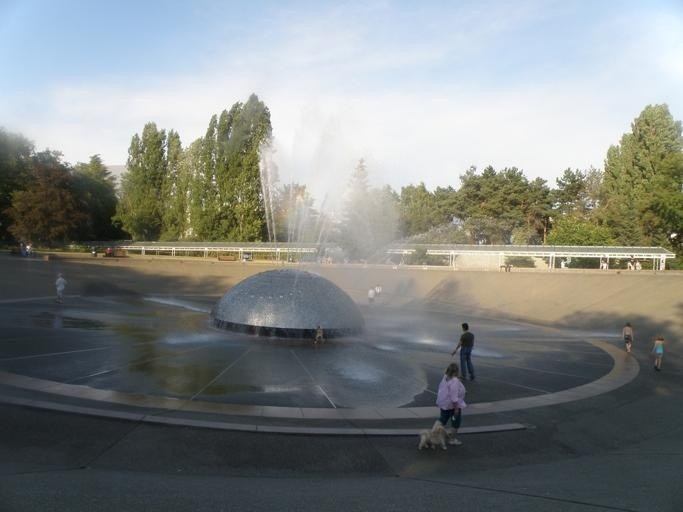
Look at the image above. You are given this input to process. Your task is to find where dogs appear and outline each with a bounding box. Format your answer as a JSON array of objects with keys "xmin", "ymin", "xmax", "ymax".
[{"xmin": 418, "ymin": 419, "xmax": 452, "ymax": 451}]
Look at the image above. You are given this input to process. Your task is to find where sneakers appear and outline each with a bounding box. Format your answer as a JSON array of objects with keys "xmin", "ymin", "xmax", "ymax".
[{"xmin": 449, "ymin": 439, "xmax": 462, "ymax": 445}]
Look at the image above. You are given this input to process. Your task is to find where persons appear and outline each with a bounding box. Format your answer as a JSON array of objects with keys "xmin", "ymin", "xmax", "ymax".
[
  {"xmin": 650, "ymin": 335, "xmax": 665, "ymax": 372},
  {"xmin": 19, "ymin": 242, "xmax": 32, "ymax": 258},
  {"xmin": 560, "ymin": 258, "xmax": 568, "ymax": 269},
  {"xmin": 434, "ymin": 362, "xmax": 467, "ymax": 448},
  {"xmin": 54, "ymin": 272, "xmax": 67, "ymax": 303},
  {"xmin": 451, "ymin": 322, "xmax": 476, "ymax": 382},
  {"xmin": 626, "ymin": 256, "xmax": 642, "ymax": 272},
  {"xmin": 503, "ymin": 256, "xmax": 512, "ymax": 273},
  {"xmin": 599, "ymin": 259, "xmax": 608, "ymax": 270},
  {"xmin": 312, "ymin": 325, "xmax": 325, "ymax": 345},
  {"xmin": 622, "ymin": 321, "xmax": 633, "ymax": 353},
  {"xmin": 367, "ymin": 284, "xmax": 383, "ymax": 308}
]
